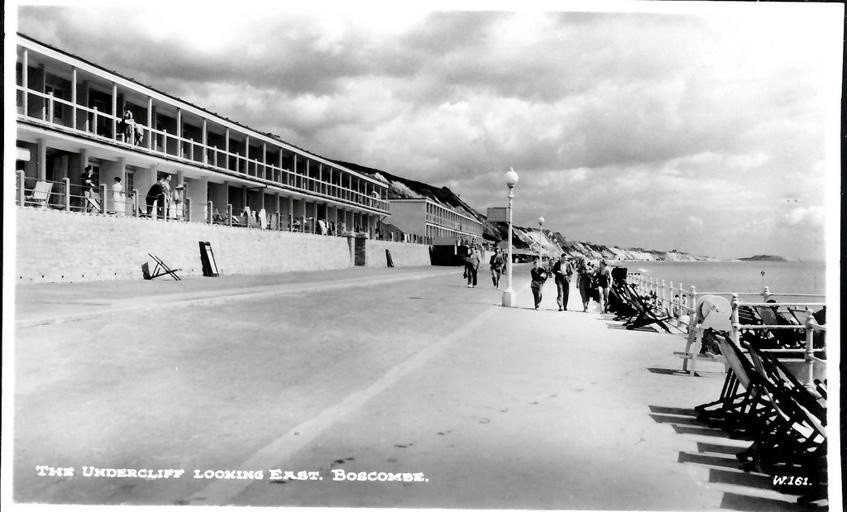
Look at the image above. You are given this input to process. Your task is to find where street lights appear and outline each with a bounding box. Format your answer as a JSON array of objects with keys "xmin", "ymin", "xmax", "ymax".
[
  {"xmin": 538, "ymin": 217, "xmax": 557, "ymax": 267},
  {"xmin": 501, "ymin": 166, "xmax": 518, "ymax": 292}
]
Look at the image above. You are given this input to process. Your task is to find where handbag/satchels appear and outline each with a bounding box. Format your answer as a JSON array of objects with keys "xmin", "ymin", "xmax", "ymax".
[{"xmin": 463, "ymin": 272, "xmax": 468, "ymax": 278}]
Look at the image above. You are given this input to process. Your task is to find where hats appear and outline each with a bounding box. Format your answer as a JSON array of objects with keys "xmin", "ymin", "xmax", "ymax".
[{"xmin": 175, "ymin": 184, "xmax": 185, "ymax": 191}]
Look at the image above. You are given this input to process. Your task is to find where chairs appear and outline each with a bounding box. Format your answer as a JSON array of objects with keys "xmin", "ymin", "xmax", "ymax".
[
  {"xmin": 85, "ymin": 197, "xmax": 115, "ymax": 213},
  {"xmin": 609, "ymin": 279, "xmax": 675, "ymax": 335},
  {"xmin": 691, "ymin": 302, "xmax": 826, "ymax": 509},
  {"xmin": 148, "ymin": 253, "xmax": 181, "ymax": 282},
  {"xmin": 25, "ymin": 180, "xmax": 53, "ymax": 208}
]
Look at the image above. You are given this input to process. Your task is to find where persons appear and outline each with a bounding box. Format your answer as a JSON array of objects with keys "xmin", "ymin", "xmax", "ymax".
[
  {"xmin": 489, "ymin": 247, "xmax": 503, "ymax": 289},
  {"xmin": 145, "ymin": 173, "xmax": 186, "ymax": 221},
  {"xmin": 530, "ymin": 253, "xmax": 613, "ymax": 315},
  {"xmin": 111, "ymin": 177, "xmax": 123, "ymax": 213},
  {"xmin": 463, "ymin": 243, "xmax": 481, "ymax": 288},
  {"xmin": 122, "ymin": 104, "xmax": 135, "ymax": 119},
  {"xmin": 81, "ymin": 168, "xmax": 96, "ymax": 211}
]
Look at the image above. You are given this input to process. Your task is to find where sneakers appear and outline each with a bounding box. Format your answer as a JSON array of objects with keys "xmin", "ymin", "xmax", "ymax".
[
  {"xmin": 535, "ymin": 306, "xmax": 606, "ymax": 314},
  {"xmin": 494, "ymin": 285, "xmax": 502, "ymax": 289},
  {"xmin": 467, "ymin": 283, "xmax": 478, "ymax": 288}
]
